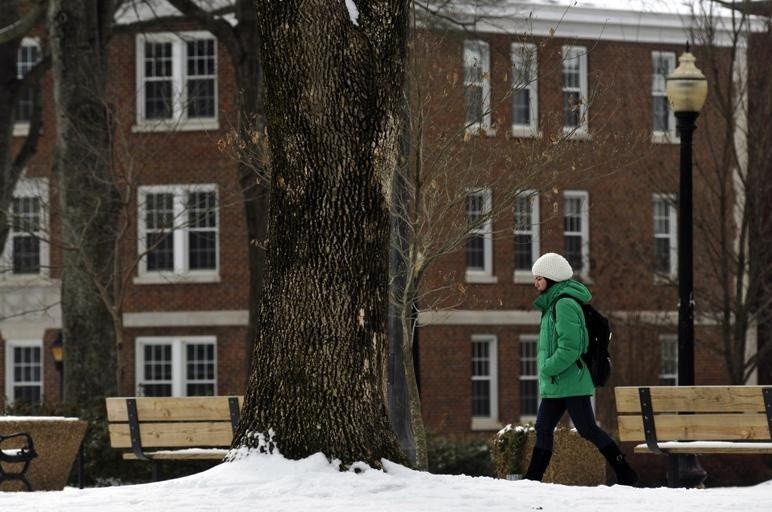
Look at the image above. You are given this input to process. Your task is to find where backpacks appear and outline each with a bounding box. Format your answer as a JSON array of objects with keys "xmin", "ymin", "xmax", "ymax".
[{"xmin": 553, "ymin": 293, "xmax": 610, "ymax": 387}]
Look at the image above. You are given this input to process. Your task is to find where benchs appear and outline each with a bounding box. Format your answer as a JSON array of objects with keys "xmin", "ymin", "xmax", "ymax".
[
  {"xmin": 105, "ymin": 395, "xmax": 245, "ymax": 481},
  {"xmin": 613, "ymin": 384, "xmax": 771, "ymax": 488}
]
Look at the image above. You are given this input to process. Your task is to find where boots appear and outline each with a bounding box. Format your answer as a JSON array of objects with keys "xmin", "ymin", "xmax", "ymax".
[
  {"xmin": 598, "ymin": 441, "xmax": 640, "ymax": 486},
  {"xmin": 522, "ymin": 446, "xmax": 552, "ymax": 480}
]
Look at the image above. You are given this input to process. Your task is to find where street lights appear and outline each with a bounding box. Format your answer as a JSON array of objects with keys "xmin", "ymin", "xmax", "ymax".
[
  {"xmin": 663, "ymin": 37, "xmax": 709, "ymax": 490},
  {"xmin": 47, "ymin": 331, "xmax": 68, "ymax": 403}
]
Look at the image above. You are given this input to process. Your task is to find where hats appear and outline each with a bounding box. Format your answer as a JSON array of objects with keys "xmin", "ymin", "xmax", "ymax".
[{"xmin": 532, "ymin": 252, "xmax": 574, "ymax": 283}]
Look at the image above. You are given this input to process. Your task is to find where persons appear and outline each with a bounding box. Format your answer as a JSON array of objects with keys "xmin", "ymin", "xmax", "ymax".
[{"xmin": 519, "ymin": 251, "xmax": 641, "ymax": 487}]
